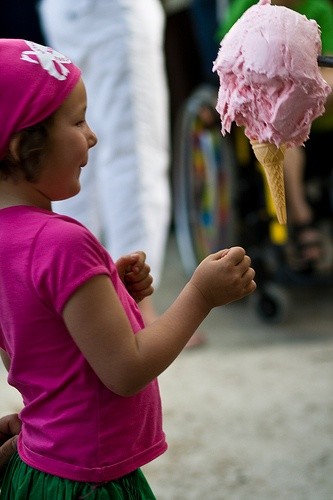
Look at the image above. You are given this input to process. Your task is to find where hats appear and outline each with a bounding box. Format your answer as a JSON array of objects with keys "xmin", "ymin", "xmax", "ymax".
[{"xmin": 0, "ymin": 38, "xmax": 81, "ymax": 153}]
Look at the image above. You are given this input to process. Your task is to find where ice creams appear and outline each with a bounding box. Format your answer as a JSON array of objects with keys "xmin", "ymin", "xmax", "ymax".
[{"xmin": 211, "ymin": 0, "xmax": 333, "ymax": 225}]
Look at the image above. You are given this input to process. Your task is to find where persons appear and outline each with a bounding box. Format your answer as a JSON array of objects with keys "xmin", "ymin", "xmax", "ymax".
[
  {"xmin": 0, "ymin": 38, "xmax": 258, "ymax": 500},
  {"xmin": 38, "ymin": 0, "xmax": 205, "ymax": 355},
  {"xmin": 0, "ymin": 413, "xmax": 23, "ymax": 467},
  {"xmin": 216, "ymin": 0, "xmax": 333, "ymax": 272}
]
